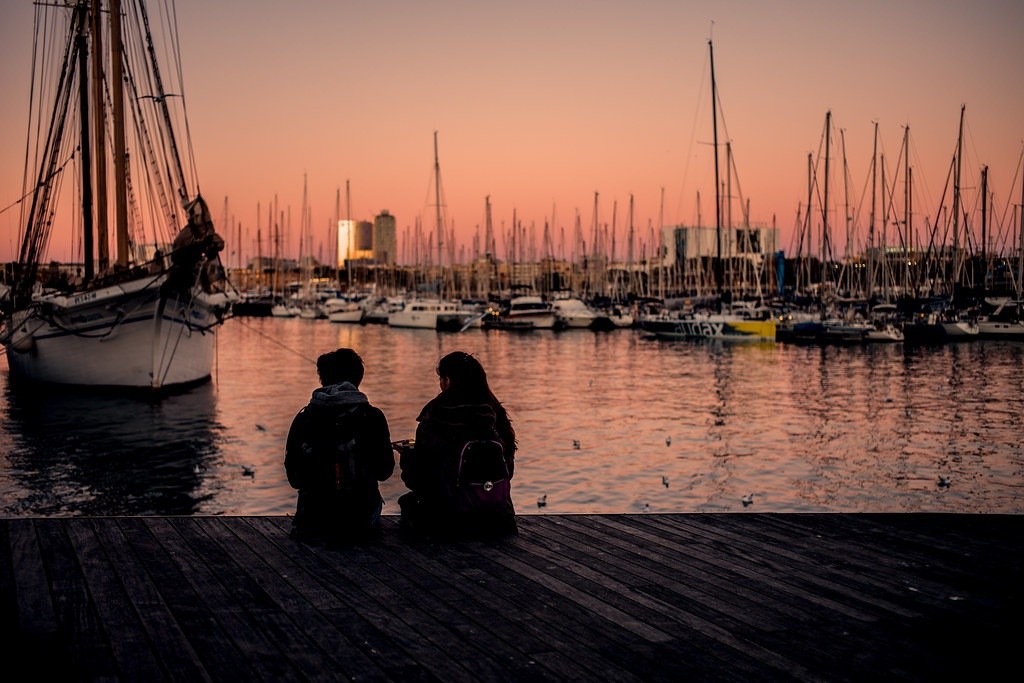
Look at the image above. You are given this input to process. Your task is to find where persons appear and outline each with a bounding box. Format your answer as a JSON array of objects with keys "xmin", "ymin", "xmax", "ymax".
[
  {"xmin": 73, "ymin": 268, "xmax": 84, "ymax": 290},
  {"xmin": 398, "ymin": 352, "xmax": 519, "ymax": 534},
  {"xmin": 284, "ymin": 348, "xmax": 395, "ymax": 535}
]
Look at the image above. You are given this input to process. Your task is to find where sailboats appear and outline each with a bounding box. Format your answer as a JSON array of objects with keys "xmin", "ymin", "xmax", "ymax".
[
  {"xmin": 114, "ymin": 20, "xmax": 1023, "ymax": 345},
  {"xmin": 0, "ymin": 0, "xmax": 247, "ymax": 402}
]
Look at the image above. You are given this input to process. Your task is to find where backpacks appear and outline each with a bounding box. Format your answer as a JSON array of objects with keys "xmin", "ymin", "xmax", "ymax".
[{"xmin": 457, "ymin": 419, "xmax": 510, "ymax": 512}]
